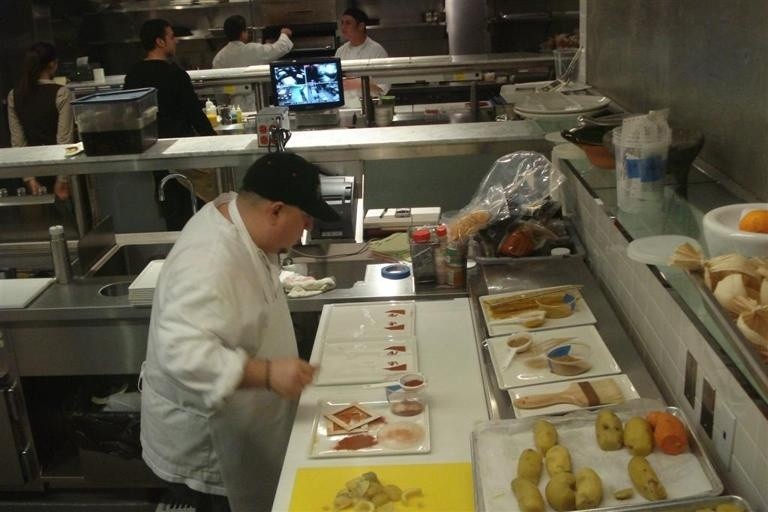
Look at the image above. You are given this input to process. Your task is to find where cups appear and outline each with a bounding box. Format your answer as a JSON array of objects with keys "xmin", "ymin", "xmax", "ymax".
[
  {"xmin": 611, "ymin": 125, "xmax": 672, "ymax": 215},
  {"xmin": 553, "ymin": 47, "xmax": 581, "ymax": 81},
  {"xmin": 93, "ymin": 68, "xmax": 105, "ymax": 82}
]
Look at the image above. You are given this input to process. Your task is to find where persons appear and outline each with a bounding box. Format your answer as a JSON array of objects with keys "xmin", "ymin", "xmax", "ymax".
[
  {"xmin": 138, "ymin": 152, "xmax": 340, "ymax": 512},
  {"xmin": 334, "ymin": 8, "xmax": 392, "ymax": 109},
  {"xmin": 122, "ymin": 19, "xmax": 218, "ymax": 137},
  {"xmin": 7, "ymin": 41, "xmax": 75, "ymax": 210},
  {"xmin": 212, "ymin": 13, "xmax": 294, "ymax": 113}
]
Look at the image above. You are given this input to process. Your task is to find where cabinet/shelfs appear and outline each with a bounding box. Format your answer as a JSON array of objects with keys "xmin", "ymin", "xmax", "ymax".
[{"xmin": 65, "ymin": 0, "xmax": 259, "ymax": 49}]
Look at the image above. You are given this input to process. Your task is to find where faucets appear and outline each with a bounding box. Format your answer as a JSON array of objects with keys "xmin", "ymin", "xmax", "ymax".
[{"xmin": 158, "ymin": 173, "xmax": 198, "ymax": 215}]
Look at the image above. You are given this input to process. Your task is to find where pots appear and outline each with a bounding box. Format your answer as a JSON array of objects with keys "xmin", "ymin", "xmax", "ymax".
[{"xmin": 560, "ymin": 125, "xmax": 705, "ymax": 171}]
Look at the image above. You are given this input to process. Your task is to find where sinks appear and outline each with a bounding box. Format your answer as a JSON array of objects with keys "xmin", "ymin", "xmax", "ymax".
[{"xmin": 91, "ymin": 243, "xmax": 173, "ymax": 275}]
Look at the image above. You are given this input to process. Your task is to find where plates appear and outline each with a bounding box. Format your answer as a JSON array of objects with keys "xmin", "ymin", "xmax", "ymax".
[
  {"xmin": 167, "ymin": 0, "xmax": 250, "ymax": 6},
  {"xmin": 478, "ymin": 285, "xmax": 642, "ymax": 418},
  {"xmin": 513, "ymin": 96, "xmax": 612, "ymax": 123},
  {"xmin": 129, "ymin": 259, "xmax": 166, "ymax": 308},
  {"xmin": 307, "ymin": 300, "xmax": 432, "ymax": 458}
]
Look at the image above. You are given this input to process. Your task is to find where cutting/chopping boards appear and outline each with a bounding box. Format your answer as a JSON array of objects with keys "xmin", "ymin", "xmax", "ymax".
[{"xmin": 286, "ymin": 463, "xmax": 476, "ymax": 512}]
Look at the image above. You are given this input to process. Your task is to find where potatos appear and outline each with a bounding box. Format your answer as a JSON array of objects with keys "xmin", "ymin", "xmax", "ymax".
[{"xmin": 512, "ymin": 410, "xmax": 667, "ymax": 511}]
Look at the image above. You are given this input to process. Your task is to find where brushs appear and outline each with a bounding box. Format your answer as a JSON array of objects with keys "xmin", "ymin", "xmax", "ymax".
[{"xmin": 513, "ymin": 378, "xmax": 626, "ymax": 409}]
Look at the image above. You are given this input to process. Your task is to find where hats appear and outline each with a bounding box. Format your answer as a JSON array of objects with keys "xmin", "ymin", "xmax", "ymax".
[{"xmin": 242, "ymin": 151, "xmax": 342, "ymax": 222}]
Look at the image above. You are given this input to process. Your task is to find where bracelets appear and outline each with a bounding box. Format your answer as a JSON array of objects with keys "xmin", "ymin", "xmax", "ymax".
[{"xmin": 265, "ymin": 359, "xmax": 272, "ymax": 391}]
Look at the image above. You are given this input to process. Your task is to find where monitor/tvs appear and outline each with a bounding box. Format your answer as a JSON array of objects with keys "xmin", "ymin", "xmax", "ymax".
[{"xmin": 269, "ymin": 57, "xmax": 346, "ymax": 113}]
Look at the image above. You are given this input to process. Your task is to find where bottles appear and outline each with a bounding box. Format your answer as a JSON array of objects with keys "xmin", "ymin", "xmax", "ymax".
[
  {"xmin": 204, "ymin": 98, "xmax": 242, "ymax": 127},
  {"xmin": 48, "ymin": 224, "xmax": 73, "ymax": 284},
  {"xmin": 409, "ymin": 224, "xmax": 466, "ymax": 288}
]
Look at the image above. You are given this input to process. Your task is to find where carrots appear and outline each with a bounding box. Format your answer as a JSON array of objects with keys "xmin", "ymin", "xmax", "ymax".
[{"xmin": 646, "ymin": 412, "xmax": 687, "ymax": 455}]
